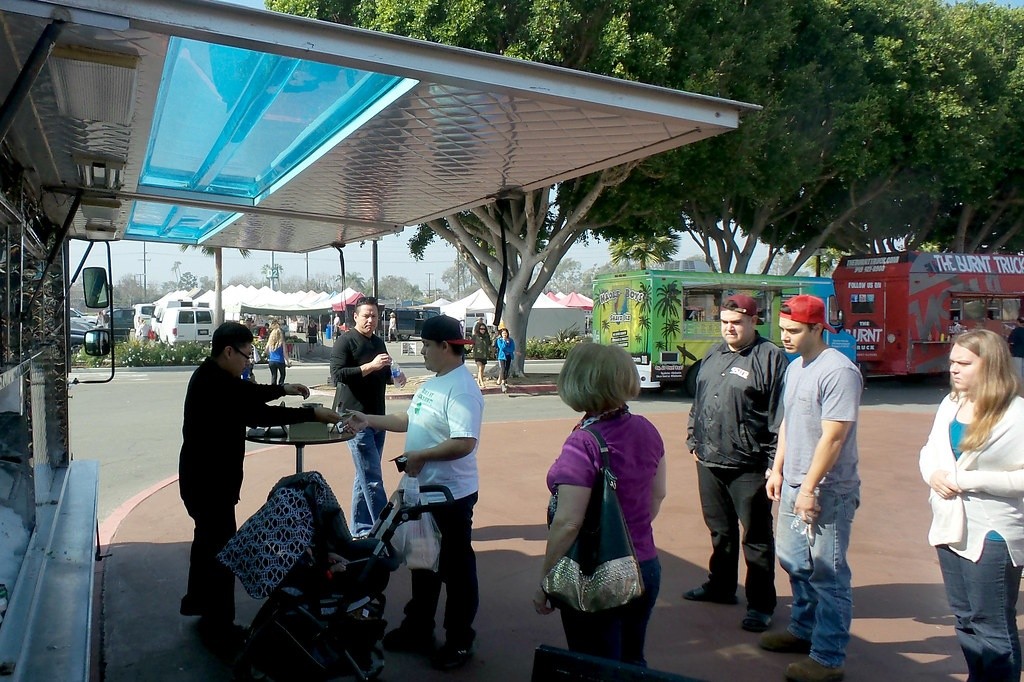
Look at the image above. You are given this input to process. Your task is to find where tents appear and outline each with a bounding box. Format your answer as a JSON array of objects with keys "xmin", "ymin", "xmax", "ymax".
[
  {"xmin": 429, "ymin": 291, "xmax": 594, "ymax": 340},
  {"xmin": 332, "ymin": 292, "xmax": 366, "ymax": 312}
]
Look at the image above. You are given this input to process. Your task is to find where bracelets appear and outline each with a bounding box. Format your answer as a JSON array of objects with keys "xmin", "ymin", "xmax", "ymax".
[{"xmin": 800, "ymin": 489, "xmax": 815, "ymax": 497}]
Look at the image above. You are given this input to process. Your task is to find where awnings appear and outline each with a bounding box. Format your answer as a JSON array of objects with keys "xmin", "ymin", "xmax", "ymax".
[
  {"xmin": 0, "ymin": 0, "xmax": 763, "ymax": 254},
  {"xmin": 686, "ymin": 282, "xmax": 806, "ymax": 291}
]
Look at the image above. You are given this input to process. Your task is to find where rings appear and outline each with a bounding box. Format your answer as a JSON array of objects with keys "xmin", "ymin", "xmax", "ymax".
[{"xmin": 536, "ymin": 608, "xmax": 541, "ymax": 612}]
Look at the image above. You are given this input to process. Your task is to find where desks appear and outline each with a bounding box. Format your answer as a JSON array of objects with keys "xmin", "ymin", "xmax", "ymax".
[{"xmin": 244, "ymin": 423, "xmax": 354, "ymax": 475}]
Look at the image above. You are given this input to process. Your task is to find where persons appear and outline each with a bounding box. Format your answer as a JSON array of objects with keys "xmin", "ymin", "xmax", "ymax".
[
  {"xmin": 681, "ymin": 295, "xmax": 789, "ymax": 632},
  {"xmin": 330, "ymin": 298, "xmax": 407, "ymax": 540},
  {"xmin": 298, "ymin": 546, "xmax": 361, "ymax": 596},
  {"xmin": 179, "ymin": 321, "xmax": 344, "ymax": 638},
  {"xmin": 91, "ymin": 340, "xmax": 110, "ymax": 355},
  {"xmin": 532, "ymin": 339, "xmax": 666, "ymax": 667},
  {"xmin": 1007, "ymin": 317, "xmax": 1024, "ymax": 384},
  {"xmin": 95, "ymin": 311, "xmax": 109, "ymax": 328},
  {"xmin": 919, "ymin": 329, "xmax": 1024, "ymax": 682},
  {"xmin": 339, "ymin": 314, "xmax": 486, "ymax": 670},
  {"xmin": 387, "ymin": 313, "xmax": 398, "ymax": 343},
  {"xmin": 241, "ymin": 317, "xmax": 341, "ymax": 385},
  {"xmin": 759, "ymin": 295, "xmax": 863, "ymax": 682},
  {"xmin": 138, "ymin": 320, "xmax": 151, "ymax": 342},
  {"xmin": 472, "ymin": 317, "xmax": 515, "ymax": 392}
]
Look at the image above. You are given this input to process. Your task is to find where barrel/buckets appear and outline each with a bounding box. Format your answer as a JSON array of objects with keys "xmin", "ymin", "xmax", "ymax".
[{"xmin": 326, "ymin": 325, "xmax": 334, "ymax": 339}]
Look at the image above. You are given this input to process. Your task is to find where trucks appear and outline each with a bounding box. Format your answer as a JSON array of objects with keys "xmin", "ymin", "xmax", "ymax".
[
  {"xmin": 590, "ymin": 273, "xmax": 835, "ymax": 399},
  {"xmin": 831, "ymin": 252, "xmax": 1023, "ymax": 378}
]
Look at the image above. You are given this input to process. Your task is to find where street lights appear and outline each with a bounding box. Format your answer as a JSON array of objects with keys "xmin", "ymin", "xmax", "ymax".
[{"xmin": 425, "ymin": 273, "xmax": 434, "ymax": 299}]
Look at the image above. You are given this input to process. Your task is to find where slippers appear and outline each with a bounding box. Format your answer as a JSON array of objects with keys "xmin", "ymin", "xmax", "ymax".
[
  {"xmin": 682, "ymin": 583, "xmax": 738, "ymax": 604},
  {"xmin": 740, "ymin": 607, "xmax": 775, "ymax": 633}
]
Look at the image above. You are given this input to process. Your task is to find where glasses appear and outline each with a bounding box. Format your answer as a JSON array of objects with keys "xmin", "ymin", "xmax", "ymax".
[
  {"xmin": 480, "ymin": 328, "xmax": 485, "ymax": 330},
  {"xmin": 356, "ymin": 296, "xmax": 378, "ymax": 305},
  {"xmin": 236, "ymin": 348, "xmax": 252, "ymax": 360}
]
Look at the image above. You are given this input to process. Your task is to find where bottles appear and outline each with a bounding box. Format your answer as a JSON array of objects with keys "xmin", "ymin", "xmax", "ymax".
[
  {"xmin": 390, "ymin": 360, "xmax": 403, "ymax": 389},
  {"xmin": 790, "ymin": 487, "xmax": 822, "ymax": 533},
  {"xmin": 403, "ymin": 474, "xmax": 420, "ymax": 509},
  {"xmin": 939, "ymin": 333, "xmax": 945, "ymax": 342}
]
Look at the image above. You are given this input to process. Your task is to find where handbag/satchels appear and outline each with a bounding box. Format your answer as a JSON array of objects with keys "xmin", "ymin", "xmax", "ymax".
[
  {"xmin": 543, "ymin": 426, "xmax": 645, "ymax": 614},
  {"xmin": 383, "ymin": 475, "xmax": 443, "ymax": 571}
]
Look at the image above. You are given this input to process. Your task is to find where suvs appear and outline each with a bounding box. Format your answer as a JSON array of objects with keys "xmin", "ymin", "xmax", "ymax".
[
  {"xmin": 69, "ymin": 304, "xmax": 212, "ymax": 349},
  {"xmin": 384, "ymin": 308, "xmax": 441, "ymax": 340}
]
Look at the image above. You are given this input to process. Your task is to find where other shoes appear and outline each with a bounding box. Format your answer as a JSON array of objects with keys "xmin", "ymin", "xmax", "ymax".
[
  {"xmin": 785, "ymin": 657, "xmax": 845, "ymax": 682},
  {"xmin": 758, "ymin": 628, "xmax": 813, "ymax": 654},
  {"xmin": 430, "ymin": 638, "xmax": 473, "ymax": 671},
  {"xmin": 382, "ymin": 627, "xmax": 437, "ymax": 652},
  {"xmin": 180, "ymin": 595, "xmax": 203, "ymax": 617},
  {"xmin": 501, "ymin": 384, "xmax": 507, "ymax": 393}
]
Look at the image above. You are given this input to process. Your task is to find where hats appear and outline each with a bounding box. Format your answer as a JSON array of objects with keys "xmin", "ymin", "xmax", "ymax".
[
  {"xmin": 720, "ymin": 294, "xmax": 764, "ymax": 326},
  {"xmin": 421, "ymin": 314, "xmax": 475, "ymax": 345},
  {"xmin": 780, "ymin": 294, "xmax": 837, "ymax": 335},
  {"xmin": 479, "ymin": 318, "xmax": 484, "ymax": 320}
]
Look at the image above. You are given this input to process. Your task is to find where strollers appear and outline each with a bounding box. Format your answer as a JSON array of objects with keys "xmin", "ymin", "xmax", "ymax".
[{"xmin": 222, "ymin": 468, "xmax": 457, "ymax": 682}]
